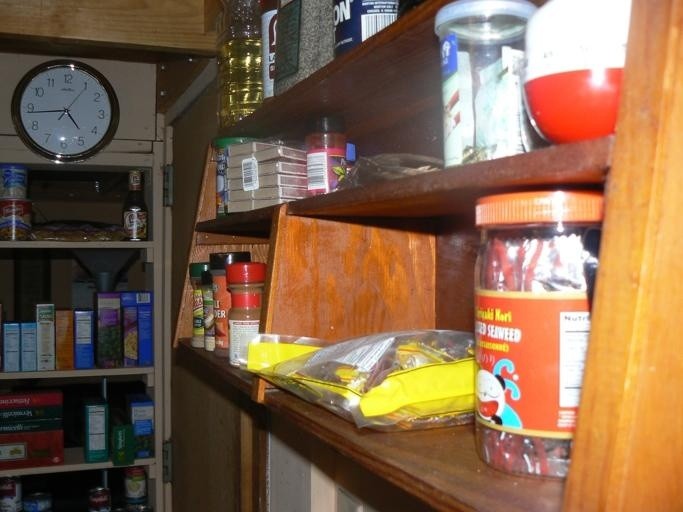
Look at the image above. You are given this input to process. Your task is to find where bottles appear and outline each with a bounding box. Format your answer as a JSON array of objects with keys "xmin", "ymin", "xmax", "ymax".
[
  {"xmin": 474, "ymin": 191, "xmax": 609, "ymax": 478},
  {"xmin": 302, "ymin": 115, "xmax": 348, "ymax": 197},
  {"xmin": 211, "ymin": 135, "xmax": 258, "ymax": 218},
  {"xmin": 188, "ymin": 251, "xmax": 268, "ymax": 369},
  {"xmin": 433, "ymin": 1, "xmax": 540, "ymax": 170},
  {"xmin": 122, "ymin": 168, "xmax": 149, "ymax": 241}
]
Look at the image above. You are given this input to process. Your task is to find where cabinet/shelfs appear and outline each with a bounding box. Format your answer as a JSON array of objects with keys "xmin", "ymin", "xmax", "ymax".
[
  {"xmin": 0, "ymin": 153, "xmax": 165, "ymax": 512},
  {"xmin": 171, "ymin": 0, "xmax": 683, "ymax": 512}
]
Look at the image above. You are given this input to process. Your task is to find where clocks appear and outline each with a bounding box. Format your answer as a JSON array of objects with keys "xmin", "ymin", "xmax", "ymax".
[{"xmin": 11, "ymin": 59, "xmax": 120, "ymax": 162}]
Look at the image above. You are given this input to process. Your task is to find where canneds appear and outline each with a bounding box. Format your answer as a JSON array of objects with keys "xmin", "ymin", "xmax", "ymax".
[
  {"xmin": 0, "ymin": 165, "xmax": 32, "ymax": 241},
  {"xmin": 88, "ymin": 487, "xmax": 112, "ymax": 512},
  {"xmin": 125, "ymin": 467, "xmax": 149, "ymax": 512},
  {"xmin": 2, "ymin": 477, "xmax": 54, "ymax": 512},
  {"xmin": 260, "ymin": 9, "xmax": 278, "ymax": 99}
]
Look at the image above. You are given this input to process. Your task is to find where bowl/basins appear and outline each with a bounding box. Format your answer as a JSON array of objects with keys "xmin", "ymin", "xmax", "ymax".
[{"xmin": 522, "ymin": 66, "xmax": 628, "ymax": 147}]
